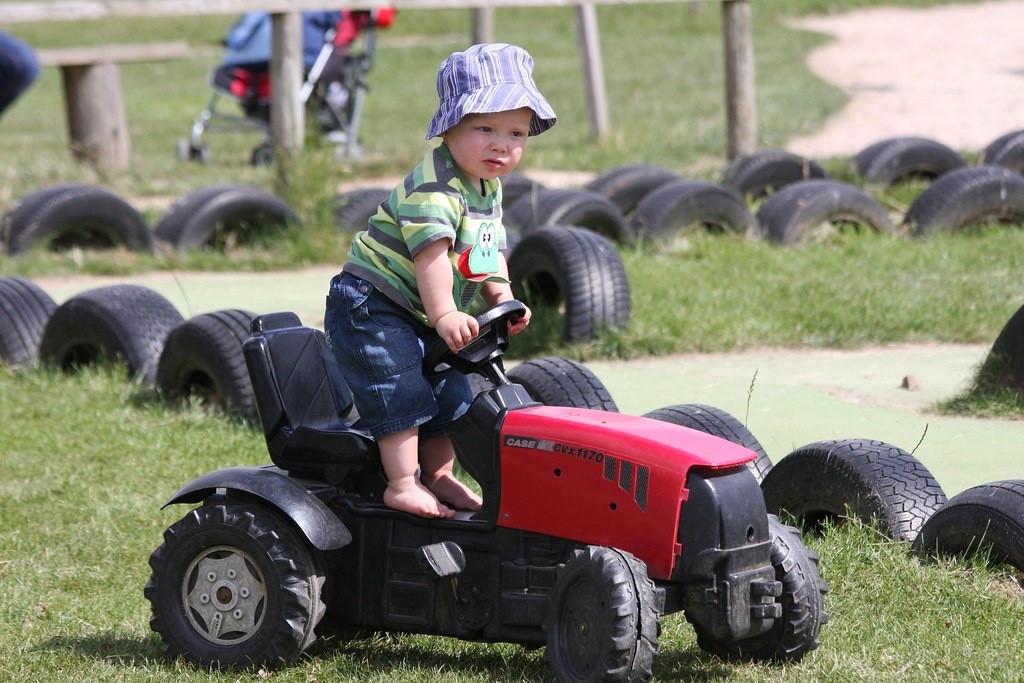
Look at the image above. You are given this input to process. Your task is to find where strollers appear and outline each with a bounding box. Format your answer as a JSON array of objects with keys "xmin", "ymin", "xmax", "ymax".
[{"xmin": 184, "ymin": 8, "xmax": 379, "ymax": 169}]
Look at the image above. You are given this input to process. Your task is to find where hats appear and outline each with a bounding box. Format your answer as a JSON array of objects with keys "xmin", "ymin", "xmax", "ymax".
[{"xmin": 425, "ymin": 43, "xmax": 557, "ymax": 140}]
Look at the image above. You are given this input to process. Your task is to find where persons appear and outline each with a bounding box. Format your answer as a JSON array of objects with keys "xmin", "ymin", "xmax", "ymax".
[
  {"xmin": 325, "ymin": 42, "xmax": 558, "ymax": 514},
  {"xmin": 0, "ymin": 25, "xmax": 39, "ymax": 116}
]
